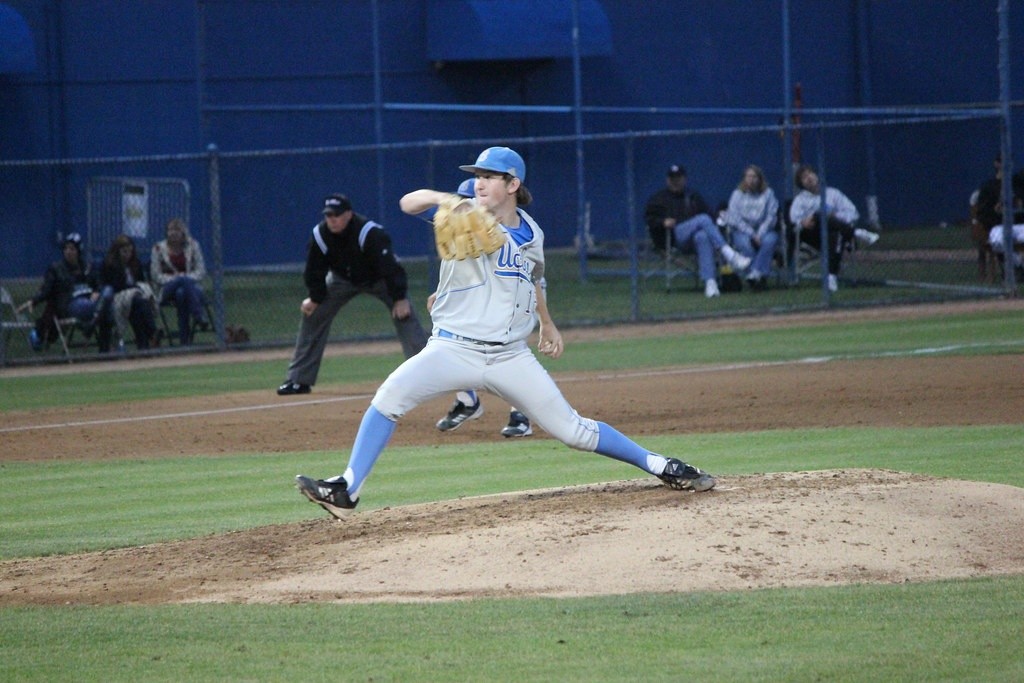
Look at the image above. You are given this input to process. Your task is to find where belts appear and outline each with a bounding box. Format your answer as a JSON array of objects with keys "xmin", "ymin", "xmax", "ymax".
[{"xmin": 439, "ymin": 329, "xmax": 503, "ymax": 347}]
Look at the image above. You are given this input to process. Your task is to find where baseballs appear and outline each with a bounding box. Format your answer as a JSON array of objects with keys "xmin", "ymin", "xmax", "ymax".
[{"xmin": 544, "ymin": 341, "xmax": 557, "ymax": 354}]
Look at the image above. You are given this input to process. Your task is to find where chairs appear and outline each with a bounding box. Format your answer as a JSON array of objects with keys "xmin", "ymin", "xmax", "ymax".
[
  {"xmin": 0, "ymin": 270, "xmax": 221, "ymax": 364},
  {"xmin": 969, "ymin": 187, "xmax": 1024, "ymax": 289},
  {"xmin": 651, "ymin": 198, "xmax": 857, "ymax": 288}
]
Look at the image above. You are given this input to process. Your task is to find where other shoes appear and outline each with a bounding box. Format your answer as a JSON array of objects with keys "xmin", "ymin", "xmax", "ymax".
[
  {"xmin": 278, "ymin": 379, "xmax": 311, "ymax": 395},
  {"xmin": 705, "ymin": 282, "xmax": 724, "ymax": 299},
  {"xmin": 729, "ymin": 252, "xmax": 751, "ymax": 274},
  {"xmin": 199, "ymin": 316, "xmax": 208, "ymax": 331},
  {"xmin": 828, "ymin": 274, "xmax": 838, "ymax": 292},
  {"xmin": 855, "ymin": 230, "xmax": 880, "ymax": 248},
  {"xmin": 151, "ymin": 325, "xmax": 166, "ymax": 347}
]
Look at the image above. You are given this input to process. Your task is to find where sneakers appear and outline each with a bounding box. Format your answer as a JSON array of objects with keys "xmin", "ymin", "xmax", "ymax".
[
  {"xmin": 436, "ymin": 396, "xmax": 485, "ymax": 432},
  {"xmin": 500, "ymin": 410, "xmax": 532, "ymax": 436},
  {"xmin": 656, "ymin": 457, "xmax": 716, "ymax": 492},
  {"xmin": 295, "ymin": 474, "xmax": 360, "ymax": 521}
]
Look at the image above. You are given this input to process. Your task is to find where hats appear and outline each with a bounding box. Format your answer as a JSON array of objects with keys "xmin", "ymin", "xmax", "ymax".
[
  {"xmin": 666, "ymin": 163, "xmax": 688, "ymax": 177},
  {"xmin": 457, "ymin": 177, "xmax": 475, "ymax": 197},
  {"xmin": 321, "ymin": 192, "xmax": 352, "ymax": 216},
  {"xmin": 63, "ymin": 232, "xmax": 84, "ymax": 256},
  {"xmin": 458, "ymin": 146, "xmax": 526, "ymax": 183}
]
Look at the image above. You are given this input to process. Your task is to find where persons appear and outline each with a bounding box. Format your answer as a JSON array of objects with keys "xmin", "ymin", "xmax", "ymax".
[
  {"xmin": 435, "ymin": 178, "xmax": 534, "ymax": 440},
  {"xmin": 645, "ymin": 163, "xmax": 751, "ymax": 300},
  {"xmin": 150, "ymin": 217, "xmax": 209, "ymax": 346},
  {"xmin": 277, "ymin": 193, "xmax": 428, "ymax": 396},
  {"xmin": 89, "ymin": 235, "xmax": 156, "ymax": 352},
  {"xmin": 714, "ymin": 165, "xmax": 780, "ymax": 293},
  {"xmin": 296, "ymin": 147, "xmax": 716, "ymax": 522},
  {"xmin": 18, "ymin": 232, "xmax": 95, "ymax": 353},
  {"xmin": 974, "ymin": 148, "xmax": 1023, "ymax": 280},
  {"xmin": 788, "ymin": 164, "xmax": 881, "ymax": 293}
]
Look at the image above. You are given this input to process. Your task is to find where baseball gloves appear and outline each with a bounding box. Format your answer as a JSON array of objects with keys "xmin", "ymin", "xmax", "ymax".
[
  {"xmin": 225, "ymin": 323, "xmax": 251, "ymax": 344},
  {"xmin": 432, "ymin": 193, "xmax": 508, "ymax": 262}
]
